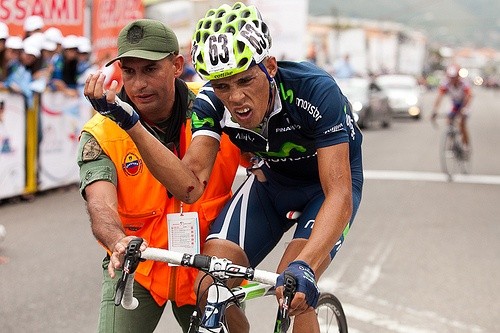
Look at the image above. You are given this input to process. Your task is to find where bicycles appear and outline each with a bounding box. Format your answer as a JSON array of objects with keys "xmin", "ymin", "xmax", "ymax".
[
  {"xmin": 113, "ymin": 210, "xmax": 349, "ymax": 333},
  {"xmin": 432, "ymin": 112, "xmax": 473, "ymax": 181}
]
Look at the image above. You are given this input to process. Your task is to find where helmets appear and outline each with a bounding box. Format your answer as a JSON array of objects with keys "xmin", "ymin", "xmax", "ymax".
[{"xmin": 190, "ymin": 2, "xmax": 272, "ymax": 81}]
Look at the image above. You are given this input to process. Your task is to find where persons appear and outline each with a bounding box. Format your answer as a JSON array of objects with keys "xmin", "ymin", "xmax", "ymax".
[
  {"xmin": 77, "ymin": 20, "xmax": 255, "ymax": 333},
  {"xmin": 83, "ymin": 1, "xmax": 363, "ymax": 333},
  {"xmin": 430, "ymin": 63, "xmax": 474, "ymax": 146},
  {"xmin": 0, "ymin": 15, "xmax": 99, "ymax": 205}
]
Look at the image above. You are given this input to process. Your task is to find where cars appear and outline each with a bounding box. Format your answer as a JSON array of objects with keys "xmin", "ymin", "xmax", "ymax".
[
  {"xmin": 337, "ymin": 78, "xmax": 392, "ymax": 129},
  {"xmin": 376, "ymin": 74, "xmax": 423, "ymax": 120},
  {"xmin": 425, "ymin": 55, "xmax": 500, "ymax": 90}
]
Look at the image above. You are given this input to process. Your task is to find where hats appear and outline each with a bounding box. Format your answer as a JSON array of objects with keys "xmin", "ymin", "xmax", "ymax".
[
  {"xmin": 105, "ymin": 18, "xmax": 179, "ymax": 68},
  {"xmin": 0, "ymin": 16, "xmax": 92, "ymax": 58}
]
